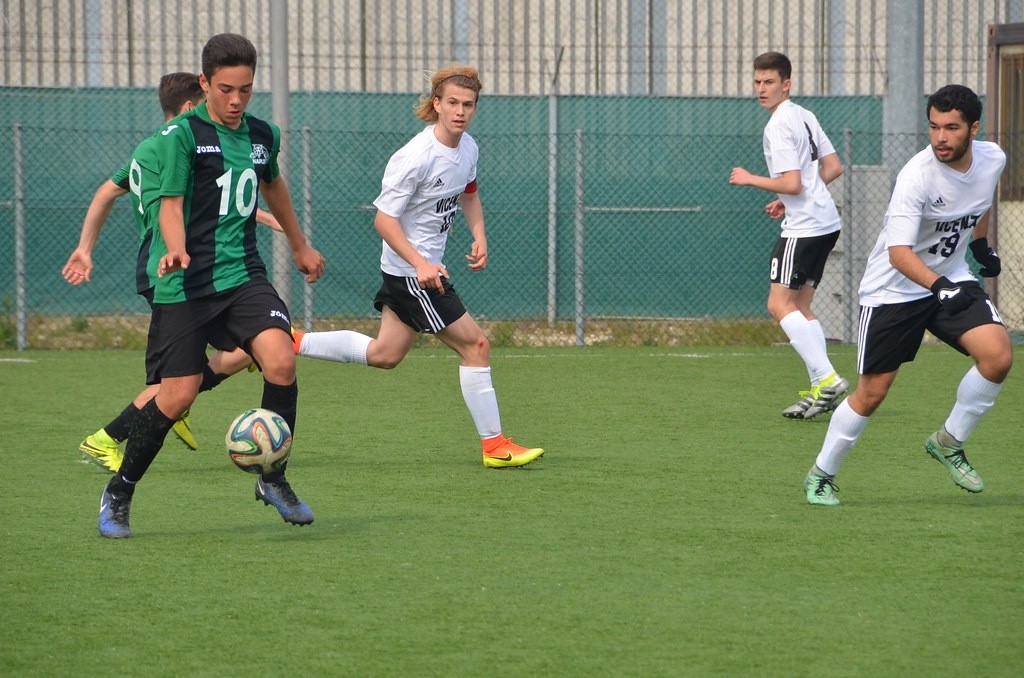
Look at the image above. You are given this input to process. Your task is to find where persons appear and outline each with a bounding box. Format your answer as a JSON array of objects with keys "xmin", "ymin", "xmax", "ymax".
[
  {"xmin": 62, "ymin": 73, "xmax": 286, "ymax": 473},
  {"xmin": 99, "ymin": 33, "xmax": 326, "ymax": 537},
  {"xmin": 730, "ymin": 52, "xmax": 848, "ymax": 418},
  {"xmin": 248, "ymin": 67, "xmax": 545, "ymax": 469},
  {"xmin": 803, "ymin": 84, "xmax": 1013, "ymax": 506}
]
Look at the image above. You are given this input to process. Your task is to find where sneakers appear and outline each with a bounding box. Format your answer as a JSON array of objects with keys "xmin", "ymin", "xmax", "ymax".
[
  {"xmin": 482, "ymin": 437, "xmax": 545, "ymax": 470},
  {"xmin": 79, "ymin": 436, "xmax": 124, "ymax": 475},
  {"xmin": 248, "ymin": 326, "xmax": 304, "ymax": 373},
  {"xmin": 99, "ymin": 476, "xmax": 135, "ymax": 539},
  {"xmin": 782, "ymin": 385, "xmax": 839, "ymax": 419},
  {"xmin": 804, "ymin": 373, "xmax": 849, "ymax": 420},
  {"xmin": 172, "ymin": 408, "xmax": 199, "ymax": 451},
  {"xmin": 255, "ymin": 474, "xmax": 314, "ymax": 526},
  {"xmin": 803, "ymin": 470, "xmax": 840, "ymax": 505},
  {"xmin": 926, "ymin": 431, "xmax": 984, "ymax": 493}
]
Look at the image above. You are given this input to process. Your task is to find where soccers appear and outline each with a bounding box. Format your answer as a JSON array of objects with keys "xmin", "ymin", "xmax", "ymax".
[{"xmin": 226, "ymin": 408, "xmax": 293, "ymax": 474}]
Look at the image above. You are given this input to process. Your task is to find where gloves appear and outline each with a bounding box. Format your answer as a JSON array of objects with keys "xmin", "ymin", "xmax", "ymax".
[
  {"xmin": 969, "ymin": 237, "xmax": 1001, "ymax": 277},
  {"xmin": 930, "ymin": 276, "xmax": 979, "ymax": 316}
]
